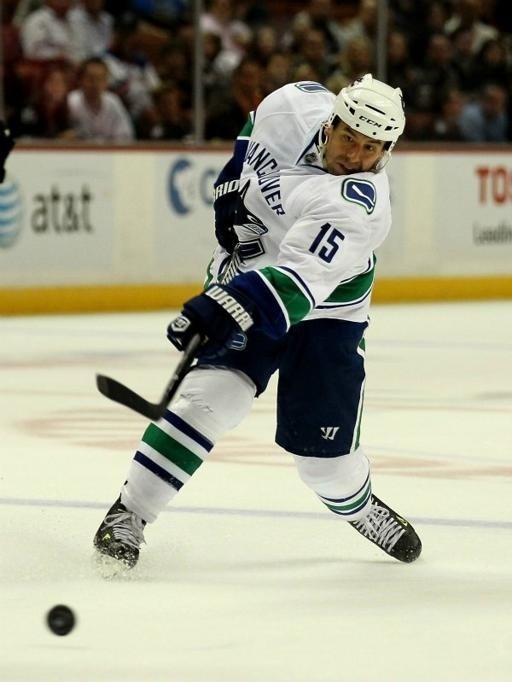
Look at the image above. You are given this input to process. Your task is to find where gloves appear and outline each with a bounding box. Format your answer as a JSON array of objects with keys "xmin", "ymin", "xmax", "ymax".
[
  {"xmin": 168, "ymin": 295, "xmax": 238, "ymax": 357},
  {"xmin": 213, "ymin": 210, "xmax": 247, "ymax": 256}
]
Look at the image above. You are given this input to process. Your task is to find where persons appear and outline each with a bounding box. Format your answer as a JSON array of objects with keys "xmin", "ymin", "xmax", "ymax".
[
  {"xmin": 1, "ymin": 0, "xmax": 512, "ymax": 150},
  {"xmin": 88, "ymin": 72, "xmax": 426, "ymax": 577}
]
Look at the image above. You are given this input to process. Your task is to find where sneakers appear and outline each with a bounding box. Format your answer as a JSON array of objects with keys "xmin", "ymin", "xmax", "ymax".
[
  {"xmin": 348, "ymin": 491, "xmax": 424, "ymax": 563},
  {"xmin": 92, "ymin": 500, "xmax": 149, "ymax": 570}
]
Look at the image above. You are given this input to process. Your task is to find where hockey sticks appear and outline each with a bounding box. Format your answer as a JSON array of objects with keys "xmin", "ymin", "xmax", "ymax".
[{"xmin": 97, "ymin": 244, "xmax": 242, "ymax": 421}]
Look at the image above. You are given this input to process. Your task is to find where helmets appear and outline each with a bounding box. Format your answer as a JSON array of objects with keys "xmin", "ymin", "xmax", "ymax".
[{"xmin": 325, "ymin": 73, "xmax": 406, "ymax": 173}]
